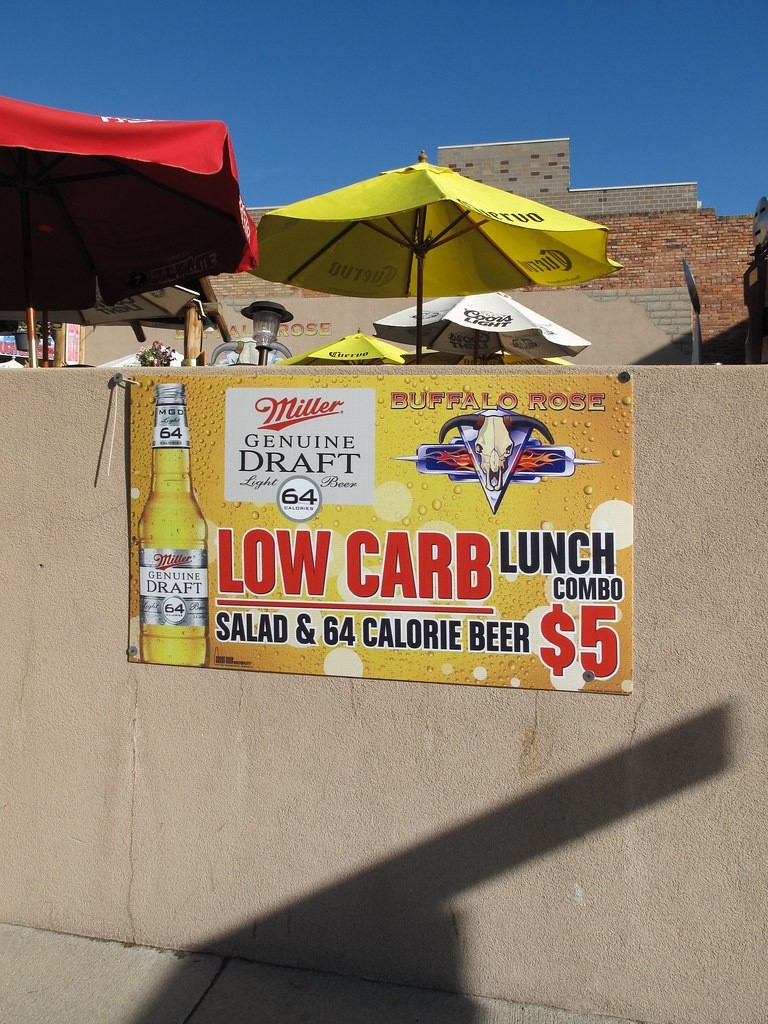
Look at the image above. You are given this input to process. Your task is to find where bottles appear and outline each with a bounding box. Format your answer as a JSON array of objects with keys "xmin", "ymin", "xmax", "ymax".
[{"xmin": 135, "ymin": 381, "xmax": 210, "ymax": 668}]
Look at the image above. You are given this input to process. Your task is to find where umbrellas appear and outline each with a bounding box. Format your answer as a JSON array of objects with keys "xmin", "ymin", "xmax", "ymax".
[
  {"xmin": 371, "ymin": 289, "xmax": 591, "ymax": 359},
  {"xmin": 0, "ymin": 93, "xmax": 262, "ymax": 368},
  {"xmin": 1, "ymin": 271, "xmax": 200, "ymax": 368},
  {"xmin": 253, "ymin": 149, "xmax": 621, "ymax": 366},
  {"xmin": 274, "ymin": 326, "xmax": 437, "ymax": 366},
  {"xmin": 400, "ymin": 342, "xmax": 580, "ymax": 365}
]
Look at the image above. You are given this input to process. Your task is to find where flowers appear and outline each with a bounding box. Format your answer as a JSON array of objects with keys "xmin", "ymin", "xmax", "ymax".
[{"xmin": 138, "ymin": 339, "xmax": 176, "ymax": 367}]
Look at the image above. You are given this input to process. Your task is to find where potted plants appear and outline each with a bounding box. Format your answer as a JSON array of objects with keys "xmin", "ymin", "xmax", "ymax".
[{"xmin": 14, "ymin": 330, "xmax": 41, "ymax": 350}]
